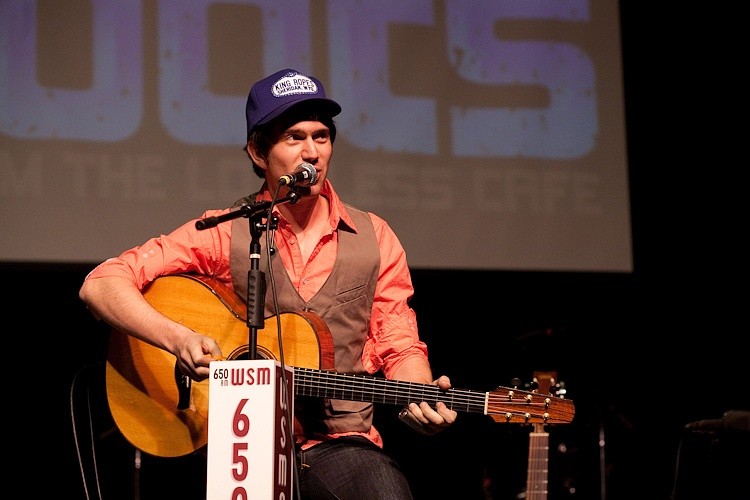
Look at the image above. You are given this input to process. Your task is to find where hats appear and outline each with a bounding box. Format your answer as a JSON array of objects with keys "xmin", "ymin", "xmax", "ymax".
[{"xmin": 245, "ymin": 69, "xmax": 342, "ymax": 143}]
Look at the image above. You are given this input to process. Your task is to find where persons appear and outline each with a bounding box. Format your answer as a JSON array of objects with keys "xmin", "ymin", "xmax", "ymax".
[{"xmin": 78, "ymin": 68, "xmax": 457, "ymax": 499}]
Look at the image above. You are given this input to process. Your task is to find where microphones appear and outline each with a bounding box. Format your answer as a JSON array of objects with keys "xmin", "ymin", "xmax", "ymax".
[
  {"xmin": 279, "ymin": 162, "xmax": 318, "ymax": 187},
  {"xmin": 684, "ymin": 410, "xmax": 750, "ymax": 432}
]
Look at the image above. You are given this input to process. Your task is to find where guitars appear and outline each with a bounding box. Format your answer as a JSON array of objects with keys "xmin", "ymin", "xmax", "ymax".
[
  {"xmin": 106, "ymin": 273, "xmax": 575, "ymax": 458},
  {"xmin": 542, "ymin": 370, "xmax": 542, "ymax": 371},
  {"xmin": 525, "ymin": 371, "xmax": 558, "ymax": 500}
]
